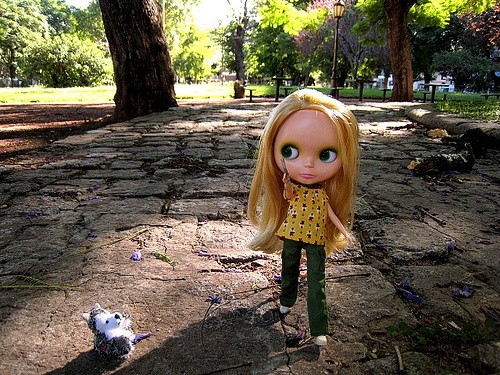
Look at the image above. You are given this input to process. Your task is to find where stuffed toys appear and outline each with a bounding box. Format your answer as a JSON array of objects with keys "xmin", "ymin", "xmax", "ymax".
[{"xmin": 82, "ymin": 302, "xmax": 151, "ymax": 358}]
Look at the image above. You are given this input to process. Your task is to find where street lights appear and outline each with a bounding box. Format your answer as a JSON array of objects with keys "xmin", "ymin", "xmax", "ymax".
[{"xmin": 330, "ymin": 0, "xmax": 345, "ymax": 97}]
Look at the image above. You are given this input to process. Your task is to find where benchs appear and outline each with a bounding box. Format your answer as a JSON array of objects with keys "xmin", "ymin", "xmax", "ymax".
[
  {"xmin": 331, "ymin": 86, "xmax": 344, "ymax": 101},
  {"xmin": 481, "ymin": 94, "xmax": 500, "ymax": 100},
  {"xmin": 420, "ymin": 91, "xmax": 431, "ymax": 102},
  {"xmin": 280, "ymin": 87, "xmax": 292, "ymax": 99},
  {"xmin": 245, "ymin": 89, "xmax": 257, "ymax": 100},
  {"xmin": 378, "ymin": 88, "xmax": 391, "ymax": 103}
]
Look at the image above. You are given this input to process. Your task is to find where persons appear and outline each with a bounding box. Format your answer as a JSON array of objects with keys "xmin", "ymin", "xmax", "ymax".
[{"xmin": 246, "ymin": 89, "xmax": 359, "ymax": 347}]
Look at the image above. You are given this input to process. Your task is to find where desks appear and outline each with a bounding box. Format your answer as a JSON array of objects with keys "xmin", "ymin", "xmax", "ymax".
[
  {"xmin": 271, "ymin": 77, "xmax": 289, "ymax": 102},
  {"xmin": 425, "ymin": 83, "xmax": 449, "ymax": 102},
  {"xmin": 349, "ymin": 79, "xmax": 377, "ymax": 101}
]
